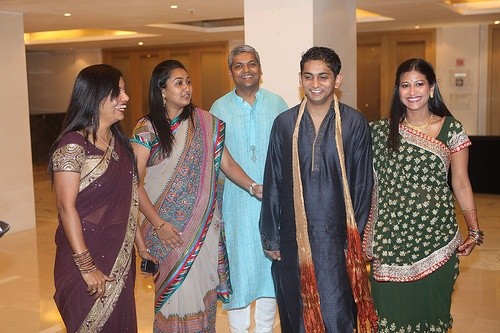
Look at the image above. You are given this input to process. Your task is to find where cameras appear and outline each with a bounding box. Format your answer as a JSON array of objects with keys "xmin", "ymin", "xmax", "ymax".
[{"xmin": 141, "ymin": 260, "xmax": 158, "ymax": 273}]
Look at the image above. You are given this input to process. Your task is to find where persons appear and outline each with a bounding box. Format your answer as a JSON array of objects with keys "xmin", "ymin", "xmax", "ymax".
[
  {"xmin": 258, "ymin": 46, "xmax": 374, "ymax": 333},
  {"xmin": 47, "ymin": 64, "xmax": 159, "ymax": 333},
  {"xmin": 368, "ymin": 57, "xmax": 479, "ymax": 333},
  {"xmin": 130, "ymin": 60, "xmax": 264, "ymax": 333},
  {"xmin": 210, "ymin": 44, "xmax": 291, "ymax": 333}
]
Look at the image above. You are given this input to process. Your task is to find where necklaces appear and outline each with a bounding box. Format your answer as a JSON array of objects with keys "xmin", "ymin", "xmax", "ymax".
[
  {"xmin": 404, "ymin": 112, "xmax": 434, "ymax": 131},
  {"xmin": 94, "ymin": 129, "xmax": 120, "ymax": 162}
]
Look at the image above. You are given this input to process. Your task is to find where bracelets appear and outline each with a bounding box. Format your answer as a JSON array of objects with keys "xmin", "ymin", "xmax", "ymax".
[
  {"xmin": 249, "ymin": 182, "xmax": 258, "ymax": 196},
  {"xmin": 151, "ymin": 219, "xmax": 166, "ymax": 231},
  {"xmin": 467, "ymin": 227, "xmax": 485, "ymax": 246},
  {"xmin": 72, "ymin": 248, "xmax": 97, "ymax": 274}
]
